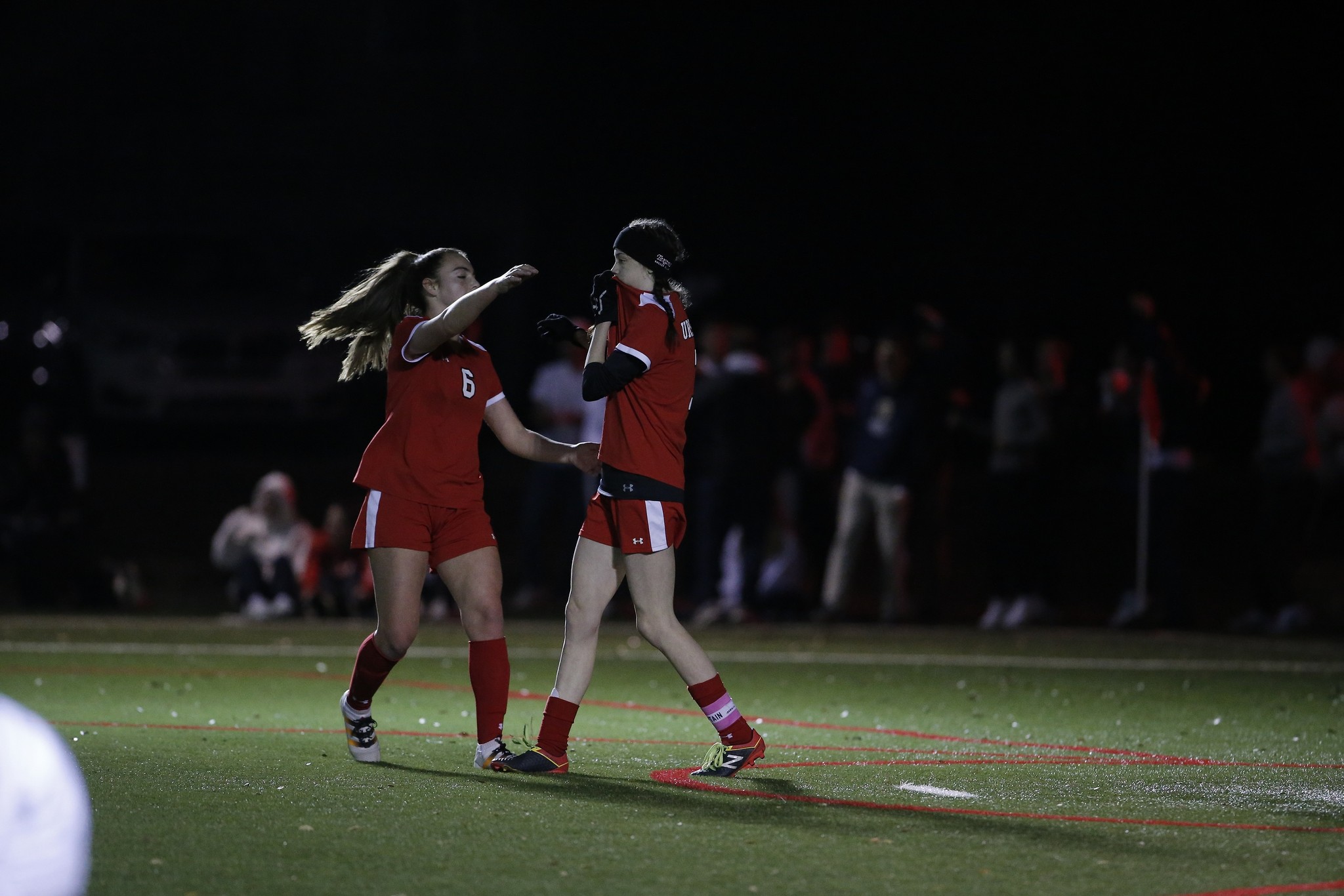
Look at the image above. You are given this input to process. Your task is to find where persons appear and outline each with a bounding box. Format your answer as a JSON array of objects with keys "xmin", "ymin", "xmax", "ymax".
[
  {"xmin": 297, "ymin": 245, "xmax": 607, "ymax": 772},
  {"xmin": 491, "ymin": 220, "xmax": 770, "ymax": 778},
  {"xmin": 203, "ymin": 299, "xmax": 1342, "ymax": 638}
]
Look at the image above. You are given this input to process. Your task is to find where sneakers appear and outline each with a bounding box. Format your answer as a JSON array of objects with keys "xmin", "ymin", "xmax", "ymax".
[
  {"xmin": 690, "ymin": 728, "xmax": 766, "ymax": 778},
  {"xmin": 340, "ymin": 690, "xmax": 381, "ymax": 762},
  {"xmin": 473, "ymin": 736, "xmax": 519, "ymax": 770},
  {"xmin": 491, "ymin": 745, "xmax": 569, "ymax": 773}
]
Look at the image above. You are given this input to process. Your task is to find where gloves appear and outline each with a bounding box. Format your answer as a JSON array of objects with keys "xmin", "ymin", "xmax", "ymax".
[
  {"xmin": 589, "ymin": 270, "xmax": 619, "ymax": 327},
  {"xmin": 538, "ymin": 314, "xmax": 586, "ymax": 348}
]
